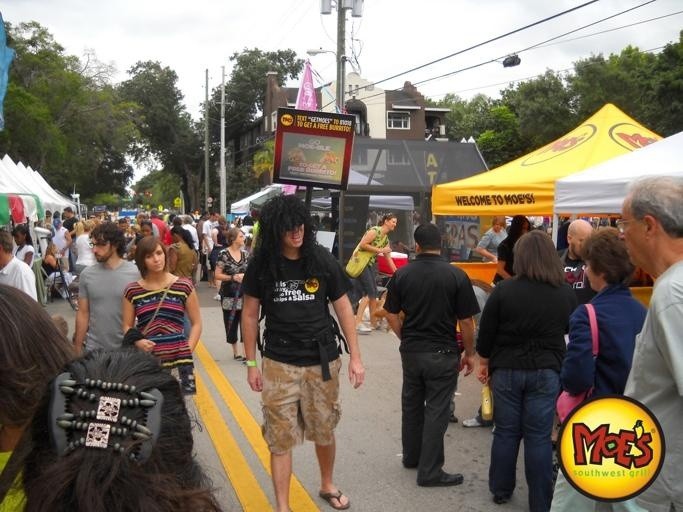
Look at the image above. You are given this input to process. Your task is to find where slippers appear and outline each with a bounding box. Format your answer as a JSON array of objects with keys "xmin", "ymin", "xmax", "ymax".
[{"xmin": 319, "ymin": 488, "xmax": 350, "ymax": 510}]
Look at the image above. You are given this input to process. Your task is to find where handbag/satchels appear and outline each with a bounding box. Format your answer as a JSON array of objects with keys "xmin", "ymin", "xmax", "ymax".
[
  {"xmin": 345, "ymin": 248, "xmax": 371, "ymax": 279},
  {"xmin": 556, "ymin": 386, "xmax": 593, "ymax": 424}
]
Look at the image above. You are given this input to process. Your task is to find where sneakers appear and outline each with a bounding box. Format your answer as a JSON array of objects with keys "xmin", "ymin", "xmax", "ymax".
[
  {"xmin": 212, "ymin": 294, "xmax": 221, "ymax": 301},
  {"xmin": 234, "ymin": 354, "xmax": 247, "ymax": 364},
  {"xmin": 493, "ymin": 495, "xmax": 511, "ymax": 503},
  {"xmin": 448, "ymin": 415, "xmax": 458, "ymax": 422},
  {"xmin": 462, "ymin": 418, "xmax": 483, "ymax": 427},
  {"xmin": 355, "ymin": 321, "xmax": 385, "ymax": 333}
]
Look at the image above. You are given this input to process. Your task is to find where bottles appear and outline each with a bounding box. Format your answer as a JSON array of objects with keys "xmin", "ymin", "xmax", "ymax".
[{"xmin": 481, "ymin": 383, "xmax": 494, "ymax": 421}]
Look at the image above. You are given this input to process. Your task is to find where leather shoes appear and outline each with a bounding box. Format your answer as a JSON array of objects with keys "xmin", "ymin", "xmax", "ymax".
[{"xmin": 404, "ymin": 462, "xmax": 463, "ymax": 486}]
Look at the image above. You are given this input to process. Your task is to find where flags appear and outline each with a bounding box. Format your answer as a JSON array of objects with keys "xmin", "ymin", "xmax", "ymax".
[{"xmin": 283, "ymin": 64, "xmax": 317, "ymax": 196}]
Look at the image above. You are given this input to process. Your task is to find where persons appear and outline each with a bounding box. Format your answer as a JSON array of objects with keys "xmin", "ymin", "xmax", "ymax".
[{"xmin": 1, "ymin": 172, "xmax": 683, "ymax": 512}]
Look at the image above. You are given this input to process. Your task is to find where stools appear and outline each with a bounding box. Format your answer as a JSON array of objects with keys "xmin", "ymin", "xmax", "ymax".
[{"xmin": 45, "ymin": 272, "xmax": 73, "ymax": 297}]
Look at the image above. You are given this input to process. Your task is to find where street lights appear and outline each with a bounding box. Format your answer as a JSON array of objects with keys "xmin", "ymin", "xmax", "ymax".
[{"xmin": 307, "ymin": 48, "xmax": 346, "ymax": 114}]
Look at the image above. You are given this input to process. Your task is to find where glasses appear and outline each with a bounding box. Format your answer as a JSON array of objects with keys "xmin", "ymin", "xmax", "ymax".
[
  {"xmin": 87, "ymin": 239, "xmax": 111, "ymax": 249},
  {"xmin": 614, "ymin": 217, "xmax": 645, "ymax": 240}
]
